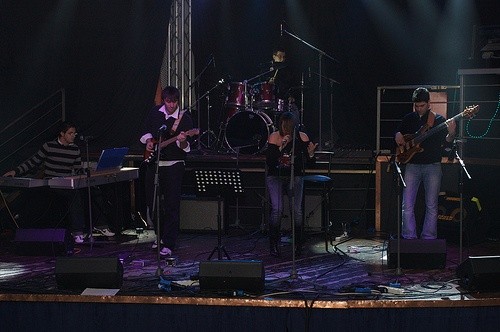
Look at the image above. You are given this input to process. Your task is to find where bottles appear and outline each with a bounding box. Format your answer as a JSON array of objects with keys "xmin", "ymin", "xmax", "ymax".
[{"xmin": 277, "ymin": 98, "xmax": 284, "ymax": 112}]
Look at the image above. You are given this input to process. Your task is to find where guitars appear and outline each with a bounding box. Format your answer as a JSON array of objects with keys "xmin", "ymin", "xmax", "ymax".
[
  {"xmin": 132, "ymin": 127, "xmax": 199, "ymax": 166},
  {"xmin": 396, "ymin": 104, "xmax": 481, "ymax": 164}
]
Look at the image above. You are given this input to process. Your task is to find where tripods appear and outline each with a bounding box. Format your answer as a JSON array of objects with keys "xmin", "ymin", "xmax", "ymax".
[
  {"xmin": 191, "ymin": 166, "xmax": 247, "ymax": 262},
  {"xmin": 189, "ymin": 79, "xmax": 227, "ymax": 152}
]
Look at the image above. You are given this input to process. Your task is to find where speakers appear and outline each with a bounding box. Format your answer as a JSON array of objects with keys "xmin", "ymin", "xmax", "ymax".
[
  {"xmin": 179, "ymin": 199, "xmax": 225, "ymax": 234},
  {"xmin": 455, "ymin": 255, "xmax": 500, "ymax": 289},
  {"xmin": 198, "ymin": 261, "xmax": 265, "ymax": 293},
  {"xmin": 54, "ymin": 256, "xmax": 125, "ymax": 291},
  {"xmin": 4, "ymin": 217, "xmax": 68, "ymax": 256},
  {"xmin": 387, "ymin": 239, "xmax": 447, "ymax": 269},
  {"xmin": 282, "ymin": 196, "xmax": 322, "ymax": 232}
]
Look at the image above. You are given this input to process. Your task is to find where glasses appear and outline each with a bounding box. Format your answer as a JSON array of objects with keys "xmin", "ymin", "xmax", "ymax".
[
  {"xmin": 275, "ymin": 54, "xmax": 286, "ymax": 59},
  {"xmin": 66, "ymin": 132, "xmax": 77, "ymax": 136}
]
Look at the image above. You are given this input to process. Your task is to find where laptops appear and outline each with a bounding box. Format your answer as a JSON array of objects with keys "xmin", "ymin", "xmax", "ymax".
[{"xmin": 84, "ymin": 147, "xmax": 128, "ymax": 171}]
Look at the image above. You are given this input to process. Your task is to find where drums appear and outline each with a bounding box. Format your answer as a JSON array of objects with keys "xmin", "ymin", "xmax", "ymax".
[
  {"xmin": 221, "ymin": 97, "xmax": 242, "ymax": 122},
  {"xmin": 224, "ymin": 110, "xmax": 274, "ymax": 156},
  {"xmin": 269, "ymin": 98, "xmax": 289, "ymax": 117},
  {"xmin": 225, "ymin": 82, "xmax": 251, "ymax": 107},
  {"xmin": 252, "ymin": 81, "xmax": 278, "ymax": 110}
]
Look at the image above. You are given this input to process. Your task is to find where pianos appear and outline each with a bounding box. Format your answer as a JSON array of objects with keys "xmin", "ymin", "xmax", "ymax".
[{"xmin": 0, "ymin": 167, "xmax": 142, "ymax": 246}]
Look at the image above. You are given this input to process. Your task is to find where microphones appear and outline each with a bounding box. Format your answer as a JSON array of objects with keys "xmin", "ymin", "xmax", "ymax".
[
  {"xmin": 159, "ymin": 124, "xmax": 168, "ymax": 131},
  {"xmin": 280, "ymin": 23, "xmax": 283, "ymax": 37},
  {"xmin": 80, "ymin": 135, "xmax": 98, "ymax": 141}
]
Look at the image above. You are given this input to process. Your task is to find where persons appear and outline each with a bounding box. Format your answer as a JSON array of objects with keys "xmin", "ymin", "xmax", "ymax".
[
  {"xmin": 4, "ymin": 121, "xmax": 88, "ymax": 244},
  {"xmin": 136, "ymin": 86, "xmax": 197, "ymax": 253},
  {"xmin": 396, "ymin": 87, "xmax": 457, "ymax": 240},
  {"xmin": 265, "ymin": 48, "xmax": 299, "ymax": 121},
  {"xmin": 265, "ymin": 112, "xmax": 319, "ymax": 254}
]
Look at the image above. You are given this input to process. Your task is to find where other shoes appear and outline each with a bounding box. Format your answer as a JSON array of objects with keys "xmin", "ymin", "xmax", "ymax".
[
  {"xmin": 390, "ymin": 233, "xmax": 405, "ymax": 239},
  {"xmin": 93, "ymin": 225, "xmax": 115, "ymax": 236},
  {"xmin": 151, "ymin": 240, "xmax": 162, "ymax": 248},
  {"xmin": 71, "ymin": 231, "xmax": 84, "ymax": 243},
  {"xmin": 160, "ymin": 247, "xmax": 173, "ymax": 255},
  {"xmin": 269, "ymin": 239, "xmax": 279, "ymax": 256},
  {"xmin": 295, "ymin": 241, "xmax": 305, "ymax": 255}
]
